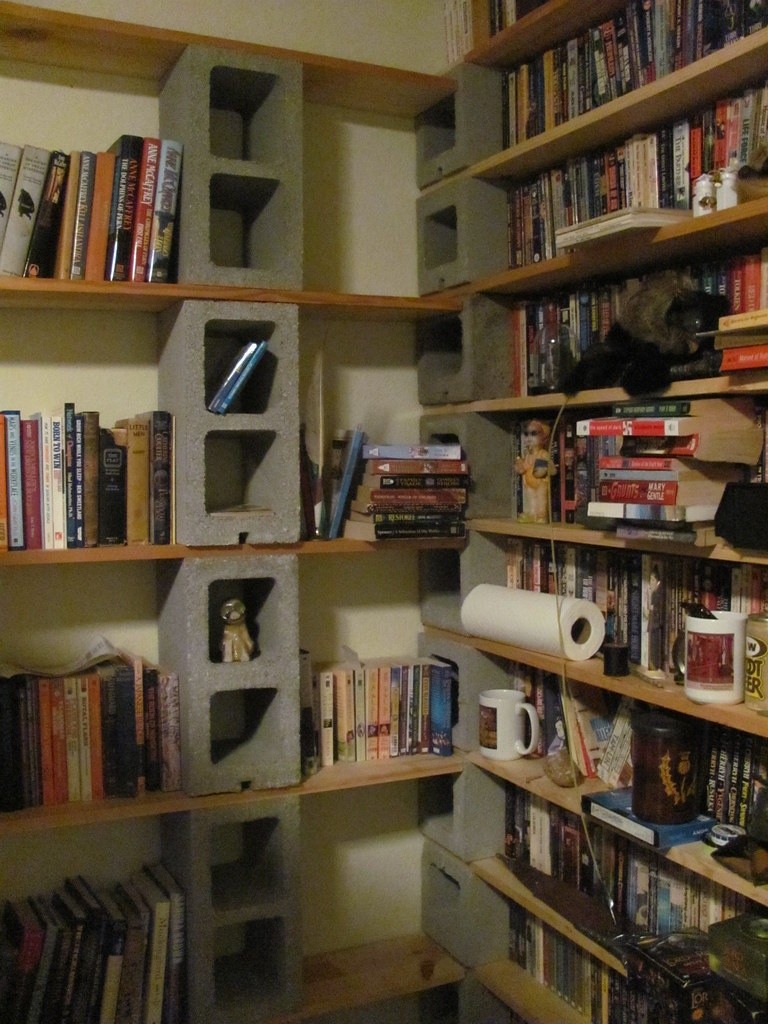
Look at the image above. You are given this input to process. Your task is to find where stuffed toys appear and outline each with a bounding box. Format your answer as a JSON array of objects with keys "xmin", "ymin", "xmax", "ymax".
[{"xmin": 555, "ymin": 281, "xmax": 731, "ymax": 395}]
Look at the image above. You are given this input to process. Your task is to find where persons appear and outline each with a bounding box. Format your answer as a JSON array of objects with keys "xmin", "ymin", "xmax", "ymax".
[{"xmin": 515, "ymin": 419, "xmax": 558, "ymax": 524}]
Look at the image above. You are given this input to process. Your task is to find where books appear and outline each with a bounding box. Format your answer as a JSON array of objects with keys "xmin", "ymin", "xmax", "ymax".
[
  {"xmin": 501, "ymin": 0, "xmax": 768, "ymax": 152},
  {"xmin": 210, "ymin": 504, "xmax": 275, "ymax": 516},
  {"xmin": 512, "ymin": 244, "xmax": 768, "ymax": 392},
  {"xmin": 0, "ymin": 402, "xmax": 178, "ymax": 554},
  {"xmin": 0, "ymin": 639, "xmax": 185, "ymax": 812},
  {"xmin": 206, "ymin": 338, "xmax": 269, "ymax": 417},
  {"xmin": 502, "ymin": 663, "xmax": 768, "ymax": 1024},
  {"xmin": 299, "ymin": 649, "xmax": 455, "ymax": 776},
  {"xmin": 0, "ymin": 859, "xmax": 187, "ymax": 1024},
  {"xmin": 512, "ymin": 398, "xmax": 768, "ymax": 548},
  {"xmin": 0, "ymin": 133, "xmax": 185, "ymax": 283},
  {"xmin": 301, "ymin": 347, "xmax": 476, "ymax": 543},
  {"xmin": 504, "ymin": 534, "xmax": 768, "ymax": 686},
  {"xmin": 484, "ymin": 0, "xmax": 547, "ymax": 37},
  {"xmin": 506, "ymin": 84, "xmax": 768, "ymax": 270}
]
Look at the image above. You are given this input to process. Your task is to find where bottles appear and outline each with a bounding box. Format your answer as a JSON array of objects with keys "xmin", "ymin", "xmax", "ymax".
[
  {"xmin": 693, "ymin": 175, "xmax": 715, "ymax": 216},
  {"xmin": 715, "ymin": 173, "xmax": 737, "ymax": 212}
]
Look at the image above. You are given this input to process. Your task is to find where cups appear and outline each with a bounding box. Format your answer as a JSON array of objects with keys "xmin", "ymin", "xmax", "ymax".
[
  {"xmin": 631, "ymin": 707, "xmax": 702, "ymax": 825},
  {"xmin": 478, "ymin": 689, "xmax": 539, "ymax": 761},
  {"xmin": 684, "ymin": 611, "xmax": 749, "ymax": 706}
]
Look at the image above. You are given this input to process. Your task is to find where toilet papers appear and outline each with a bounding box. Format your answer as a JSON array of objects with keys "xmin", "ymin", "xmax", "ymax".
[{"xmin": 460, "ymin": 583, "xmax": 605, "ymax": 663}]
